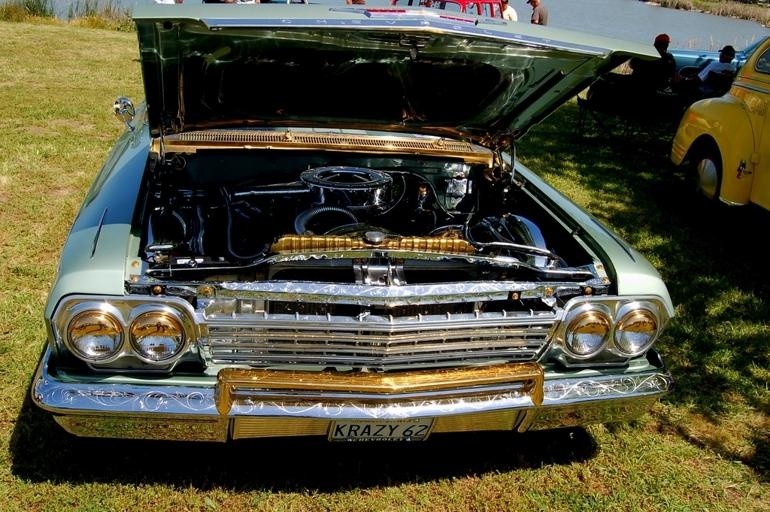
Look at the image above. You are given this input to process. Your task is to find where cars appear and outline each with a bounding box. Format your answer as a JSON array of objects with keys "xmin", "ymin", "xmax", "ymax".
[
  {"xmin": 15, "ymin": 1, "xmax": 679, "ymax": 454},
  {"xmin": 668, "ymin": 38, "xmax": 770, "ymax": 216},
  {"xmin": 663, "ymin": 30, "xmax": 770, "ymax": 76}
]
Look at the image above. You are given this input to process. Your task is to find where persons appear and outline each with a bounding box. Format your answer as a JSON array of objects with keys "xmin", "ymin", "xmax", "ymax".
[
  {"xmin": 526, "ymin": 0, "xmax": 548, "ymax": 26},
  {"xmin": 494, "ymin": 0, "xmax": 517, "ymax": 22},
  {"xmin": 601, "ymin": 34, "xmax": 678, "ymax": 94},
  {"xmin": 673, "ymin": 44, "xmax": 739, "ymax": 94}
]
